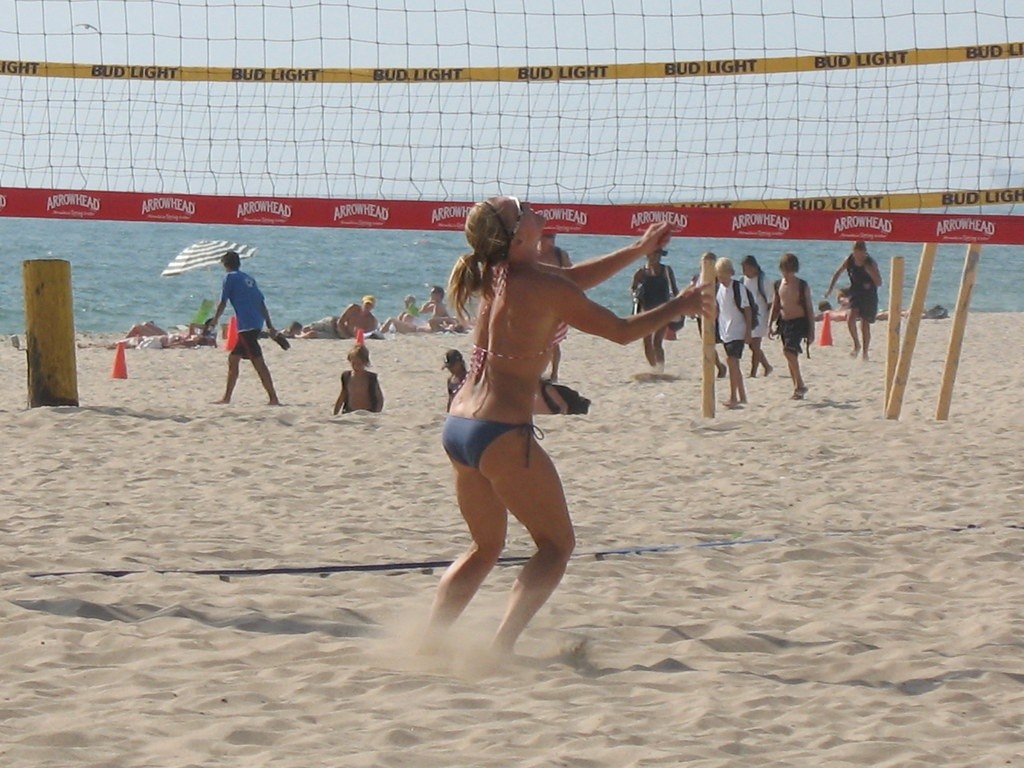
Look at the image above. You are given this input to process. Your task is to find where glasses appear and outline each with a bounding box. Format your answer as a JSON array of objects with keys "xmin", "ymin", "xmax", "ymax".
[{"xmin": 506, "ymin": 197, "xmax": 525, "ymax": 244}]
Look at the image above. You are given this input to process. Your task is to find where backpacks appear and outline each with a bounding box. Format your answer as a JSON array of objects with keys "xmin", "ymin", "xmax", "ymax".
[{"xmin": 715, "ymin": 280, "xmax": 759, "ymax": 325}]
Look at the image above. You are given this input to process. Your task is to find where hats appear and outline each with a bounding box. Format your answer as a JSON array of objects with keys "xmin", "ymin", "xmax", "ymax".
[
  {"xmin": 440, "ymin": 350, "xmax": 462, "ymax": 370},
  {"xmin": 221, "ymin": 251, "xmax": 241, "ymax": 272},
  {"xmin": 654, "ymin": 249, "xmax": 669, "ymax": 258},
  {"xmin": 362, "ymin": 295, "xmax": 377, "ymax": 306}
]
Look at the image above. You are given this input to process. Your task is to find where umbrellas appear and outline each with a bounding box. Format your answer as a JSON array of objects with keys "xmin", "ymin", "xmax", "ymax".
[{"xmin": 160, "ymin": 239, "xmax": 260, "ymax": 313}]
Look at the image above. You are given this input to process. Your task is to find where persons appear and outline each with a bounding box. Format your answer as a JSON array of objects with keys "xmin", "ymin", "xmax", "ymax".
[
  {"xmin": 714, "ymin": 257, "xmax": 752, "ymax": 408},
  {"xmin": 824, "ymin": 239, "xmax": 882, "ymax": 362},
  {"xmin": 815, "ymin": 286, "xmax": 949, "ymax": 322},
  {"xmin": 209, "ymin": 251, "xmax": 290, "ymax": 405},
  {"xmin": 259, "ymin": 286, "xmax": 463, "ymax": 338},
  {"xmin": 675, "ymin": 253, "xmax": 778, "ymax": 379},
  {"xmin": 416, "ymin": 195, "xmax": 715, "ymax": 671},
  {"xmin": 631, "ymin": 247, "xmax": 679, "ymax": 373},
  {"xmin": 768, "ymin": 254, "xmax": 815, "ymax": 400},
  {"xmin": 334, "ymin": 345, "xmax": 384, "ymax": 412},
  {"xmin": 97, "ymin": 317, "xmax": 217, "ymax": 348},
  {"xmin": 532, "ymin": 233, "xmax": 591, "ymax": 414},
  {"xmin": 440, "ymin": 349, "xmax": 468, "ymax": 412}
]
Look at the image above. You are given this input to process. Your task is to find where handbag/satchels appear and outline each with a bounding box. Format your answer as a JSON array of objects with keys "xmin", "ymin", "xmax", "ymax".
[{"xmin": 668, "ymin": 293, "xmax": 680, "ymax": 323}]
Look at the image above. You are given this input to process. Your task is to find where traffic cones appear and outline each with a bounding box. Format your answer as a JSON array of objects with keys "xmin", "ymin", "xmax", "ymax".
[
  {"xmin": 111, "ymin": 342, "xmax": 128, "ymax": 379},
  {"xmin": 356, "ymin": 329, "xmax": 365, "ymax": 344},
  {"xmin": 225, "ymin": 315, "xmax": 237, "ymax": 351},
  {"xmin": 819, "ymin": 311, "xmax": 833, "ymax": 346}
]
